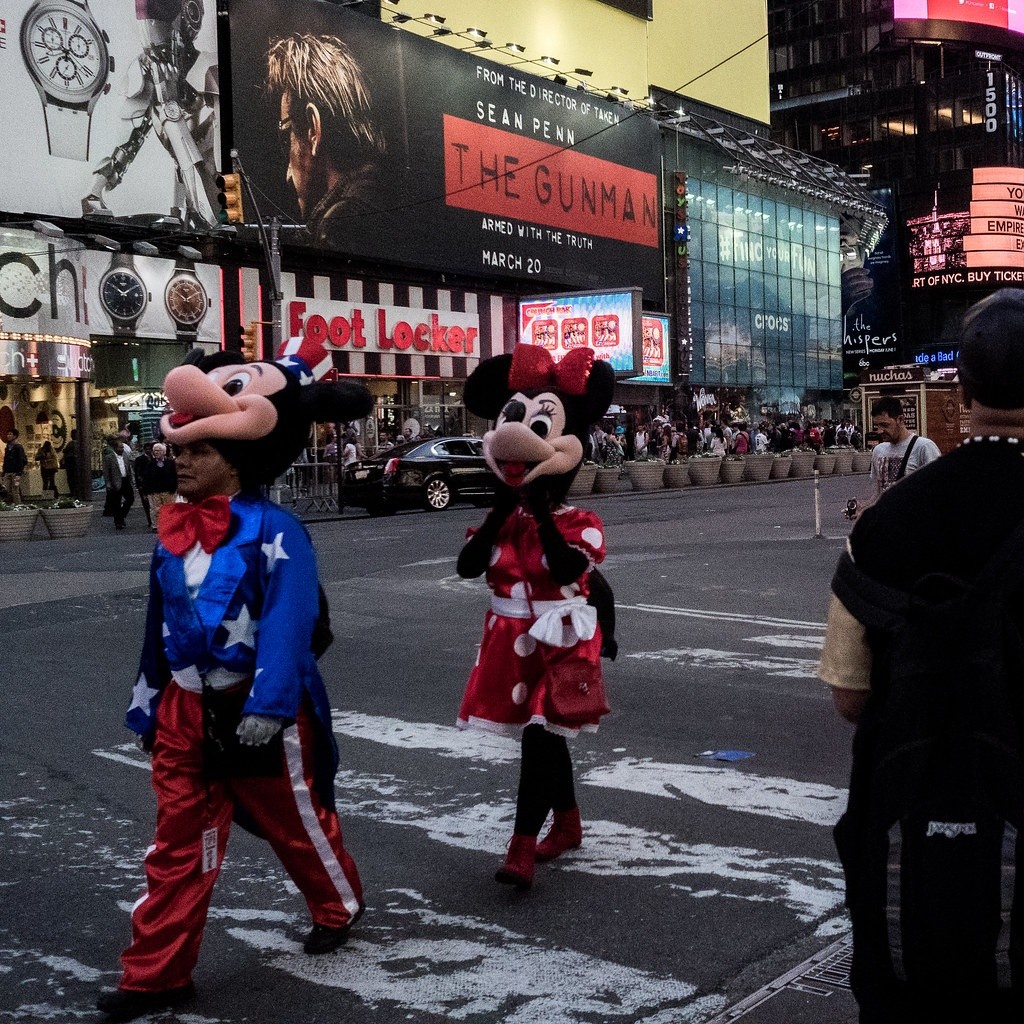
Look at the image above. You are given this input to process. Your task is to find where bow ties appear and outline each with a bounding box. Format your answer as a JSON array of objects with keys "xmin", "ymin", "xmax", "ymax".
[{"xmin": 156, "ymin": 495, "xmax": 232, "ymax": 557}]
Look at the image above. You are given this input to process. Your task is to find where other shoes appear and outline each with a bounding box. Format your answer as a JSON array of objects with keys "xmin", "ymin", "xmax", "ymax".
[
  {"xmin": 103, "ymin": 509, "xmax": 114, "ymax": 517},
  {"xmin": 116, "ymin": 519, "xmax": 126, "ymax": 529},
  {"xmin": 97, "ymin": 981, "xmax": 195, "ymax": 1014},
  {"xmin": 304, "ymin": 903, "xmax": 366, "ymax": 954},
  {"xmin": 153, "ymin": 528, "xmax": 157, "ymax": 533}
]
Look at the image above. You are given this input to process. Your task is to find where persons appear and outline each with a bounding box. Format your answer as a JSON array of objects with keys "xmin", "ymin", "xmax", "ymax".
[
  {"xmin": 35, "ymin": 441, "xmax": 59, "ymax": 490},
  {"xmin": 62, "ymin": 428, "xmax": 88, "ymax": 500},
  {"xmin": 819, "ymin": 286, "xmax": 1024, "ymax": 1024},
  {"xmin": 840, "ymin": 396, "xmax": 941, "ymax": 520},
  {"xmin": 376, "ymin": 426, "xmax": 435, "ymax": 453},
  {"xmin": 0, "ymin": 429, "xmax": 27, "ymax": 504},
  {"xmin": 299, "ymin": 423, "xmax": 369, "ymax": 483},
  {"xmin": 587, "ymin": 405, "xmax": 863, "ymax": 465},
  {"xmin": 102, "ymin": 429, "xmax": 178, "ymax": 533},
  {"xmin": 267, "ymin": 32, "xmax": 392, "ymax": 255},
  {"xmin": 99, "ymin": 349, "xmax": 379, "ymax": 1015}
]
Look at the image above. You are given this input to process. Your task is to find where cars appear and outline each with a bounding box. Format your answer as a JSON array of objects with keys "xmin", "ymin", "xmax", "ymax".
[{"xmin": 341, "ymin": 436, "xmax": 505, "ymax": 515}]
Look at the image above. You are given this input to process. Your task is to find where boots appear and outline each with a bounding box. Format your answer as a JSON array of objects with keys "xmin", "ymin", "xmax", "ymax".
[
  {"xmin": 534, "ymin": 807, "xmax": 582, "ymax": 858},
  {"xmin": 495, "ymin": 833, "xmax": 536, "ymax": 888}
]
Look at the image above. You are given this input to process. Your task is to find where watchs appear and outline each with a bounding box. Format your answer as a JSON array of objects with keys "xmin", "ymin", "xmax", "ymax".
[
  {"xmin": 99, "ymin": 253, "xmax": 152, "ymax": 337},
  {"xmin": 164, "ymin": 259, "xmax": 211, "ymax": 340},
  {"xmin": 159, "ymin": 101, "xmax": 184, "ymax": 123},
  {"xmin": 20, "ymin": 0, "xmax": 115, "ymax": 163}
]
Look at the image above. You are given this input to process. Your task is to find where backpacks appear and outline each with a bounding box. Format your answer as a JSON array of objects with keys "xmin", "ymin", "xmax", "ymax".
[{"xmin": 677, "ymin": 433, "xmax": 688, "ymax": 454}]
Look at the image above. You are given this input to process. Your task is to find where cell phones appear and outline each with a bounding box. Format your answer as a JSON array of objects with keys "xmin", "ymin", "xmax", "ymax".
[{"xmin": 847, "ymin": 500, "xmax": 857, "ymax": 518}]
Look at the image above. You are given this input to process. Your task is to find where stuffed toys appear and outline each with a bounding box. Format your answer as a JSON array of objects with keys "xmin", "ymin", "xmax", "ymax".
[{"xmin": 456, "ymin": 345, "xmax": 616, "ymax": 882}]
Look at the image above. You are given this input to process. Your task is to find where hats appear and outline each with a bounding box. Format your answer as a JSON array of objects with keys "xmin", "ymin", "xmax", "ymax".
[
  {"xmin": 959, "ymin": 288, "xmax": 1024, "ymax": 412},
  {"xmin": 396, "ymin": 435, "xmax": 406, "ymax": 441}
]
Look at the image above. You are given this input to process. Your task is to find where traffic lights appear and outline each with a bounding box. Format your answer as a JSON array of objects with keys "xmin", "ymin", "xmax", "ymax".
[
  {"xmin": 216, "ymin": 174, "xmax": 243, "ymax": 224},
  {"xmin": 240, "ymin": 323, "xmax": 258, "ymax": 361}
]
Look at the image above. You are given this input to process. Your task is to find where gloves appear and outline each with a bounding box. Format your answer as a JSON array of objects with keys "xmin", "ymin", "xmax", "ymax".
[
  {"xmin": 134, "ymin": 733, "xmax": 151, "ymax": 755},
  {"xmin": 236, "ymin": 716, "xmax": 282, "ymax": 746}
]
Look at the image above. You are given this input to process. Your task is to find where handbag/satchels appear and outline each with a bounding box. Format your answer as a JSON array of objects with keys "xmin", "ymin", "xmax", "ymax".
[
  {"xmin": 617, "ymin": 445, "xmax": 625, "ymax": 455},
  {"xmin": 747, "ymin": 444, "xmax": 751, "ymax": 452}
]
